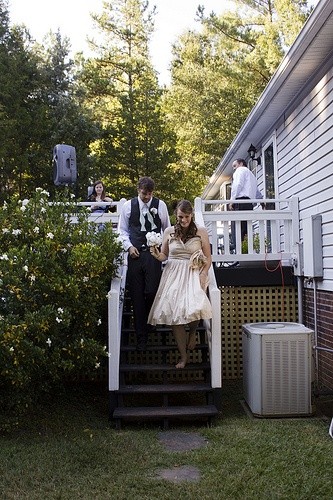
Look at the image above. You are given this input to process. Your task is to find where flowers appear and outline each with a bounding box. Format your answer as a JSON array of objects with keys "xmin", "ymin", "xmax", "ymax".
[
  {"xmin": 151, "ymin": 207, "xmax": 157, "ymax": 215},
  {"xmin": 145, "ymin": 231, "xmax": 163, "ymax": 247}
]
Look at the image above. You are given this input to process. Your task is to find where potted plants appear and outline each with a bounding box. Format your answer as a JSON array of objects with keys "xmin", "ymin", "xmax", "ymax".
[{"xmin": 238, "ymin": 233, "xmax": 281, "ymax": 268}]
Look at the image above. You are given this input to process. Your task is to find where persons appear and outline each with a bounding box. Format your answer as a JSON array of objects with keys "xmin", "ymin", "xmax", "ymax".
[
  {"xmin": 229, "ymin": 159, "xmax": 258, "ymax": 249},
  {"xmin": 150, "ymin": 200, "xmax": 212, "ymax": 368},
  {"xmin": 89, "ymin": 180, "xmax": 113, "ymax": 213},
  {"xmin": 117, "ymin": 177, "xmax": 171, "ymax": 332}
]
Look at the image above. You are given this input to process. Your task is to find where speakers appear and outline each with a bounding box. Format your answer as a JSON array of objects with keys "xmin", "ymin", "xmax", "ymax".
[{"xmin": 51, "ymin": 144, "xmax": 78, "ymax": 186}]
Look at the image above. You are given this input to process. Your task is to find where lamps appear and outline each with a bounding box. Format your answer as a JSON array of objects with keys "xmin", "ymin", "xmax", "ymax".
[{"xmin": 247, "ymin": 144, "xmax": 261, "ymax": 164}]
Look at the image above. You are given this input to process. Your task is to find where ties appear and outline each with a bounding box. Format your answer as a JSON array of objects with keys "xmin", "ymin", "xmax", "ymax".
[{"xmin": 142, "ymin": 205, "xmax": 148, "ymax": 215}]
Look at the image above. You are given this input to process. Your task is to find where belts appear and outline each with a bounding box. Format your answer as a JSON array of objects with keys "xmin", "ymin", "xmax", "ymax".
[{"xmin": 141, "ymin": 248, "xmax": 148, "ymax": 252}]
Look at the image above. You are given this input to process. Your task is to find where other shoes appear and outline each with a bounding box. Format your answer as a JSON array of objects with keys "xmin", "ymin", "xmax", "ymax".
[
  {"xmin": 188, "ymin": 331, "xmax": 196, "ymax": 349},
  {"xmin": 176, "ymin": 355, "xmax": 189, "ymax": 368},
  {"xmin": 136, "ymin": 340, "xmax": 146, "ymax": 353}
]
